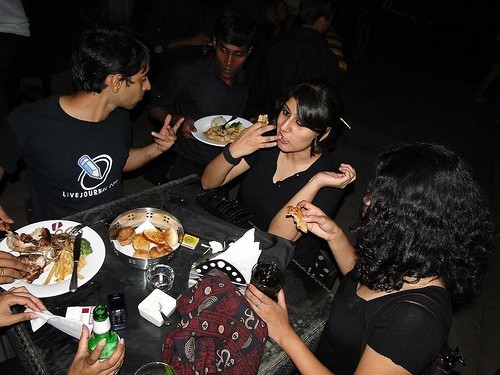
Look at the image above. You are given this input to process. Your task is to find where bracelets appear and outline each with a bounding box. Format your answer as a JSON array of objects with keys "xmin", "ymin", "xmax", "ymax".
[{"xmin": 223, "ymin": 143, "xmax": 242, "ymax": 166}]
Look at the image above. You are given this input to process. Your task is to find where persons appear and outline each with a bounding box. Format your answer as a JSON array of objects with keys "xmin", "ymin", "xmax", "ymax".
[
  {"xmin": 245, "ymin": 134, "xmax": 500, "ymax": 375},
  {"xmin": 0, "ymin": 0, "xmax": 344, "ymax": 121},
  {"xmin": 0, "ymin": 285, "xmax": 126, "ymax": 375},
  {"xmin": 201, "ymin": 79, "xmax": 356, "ymax": 270},
  {"xmin": 0, "ymin": 22, "xmax": 185, "ymax": 233},
  {"xmin": 0, "ymin": 251, "xmax": 32, "ymax": 285},
  {"xmin": 147, "ymin": 6, "xmax": 256, "ymax": 207}
]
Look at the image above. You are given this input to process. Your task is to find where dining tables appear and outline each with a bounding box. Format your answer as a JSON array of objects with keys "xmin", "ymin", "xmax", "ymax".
[{"xmin": 6, "ymin": 173, "xmax": 337, "ymax": 375}]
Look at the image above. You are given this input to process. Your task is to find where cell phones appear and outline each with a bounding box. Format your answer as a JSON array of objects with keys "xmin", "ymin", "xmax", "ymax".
[{"xmin": 107, "ymin": 292, "xmax": 128, "ymax": 331}]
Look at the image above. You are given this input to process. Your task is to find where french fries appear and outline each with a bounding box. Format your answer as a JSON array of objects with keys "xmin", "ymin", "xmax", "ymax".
[{"xmin": 44, "ymin": 247, "xmax": 86, "ymax": 285}]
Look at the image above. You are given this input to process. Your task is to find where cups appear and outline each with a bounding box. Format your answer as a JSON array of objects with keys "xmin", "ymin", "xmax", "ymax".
[
  {"xmin": 134, "ymin": 362, "xmax": 177, "ymax": 375},
  {"xmin": 145, "ymin": 264, "xmax": 175, "ymax": 293},
  {"xmin": 251, "ymin": 260, "xmax": 286, "ymax": 299}
]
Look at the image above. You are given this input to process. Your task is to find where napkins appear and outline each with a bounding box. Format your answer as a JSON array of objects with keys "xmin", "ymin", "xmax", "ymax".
[{"xmin": 17, "ymin": 304, "xmax": 90, "ymax": 344}]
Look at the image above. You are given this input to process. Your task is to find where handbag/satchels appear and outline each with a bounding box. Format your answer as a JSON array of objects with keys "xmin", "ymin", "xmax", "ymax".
[
  {"xmin": 361, "ymin": 293, "xmax": 466, "ymax": 375},
  {"xmin": 196, "ymin": 189, "xmax": 254, "ymax": 226},
  {"xmin": 162, "ymin": 269, "xmax": 268, "ymax": 375}
]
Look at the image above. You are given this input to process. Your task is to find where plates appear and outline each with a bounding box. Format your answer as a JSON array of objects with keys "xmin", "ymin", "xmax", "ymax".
[
  {"xmin": 191, "ymin": 115, "xmax": 255, "ymax": 147},
  {"xmin": 0, "ymin": 219, "xmax": 105, "ymax": 298}
]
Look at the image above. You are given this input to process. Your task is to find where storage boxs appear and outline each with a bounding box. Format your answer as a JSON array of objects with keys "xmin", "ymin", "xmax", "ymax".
[
  {"xmin": 181, "ymin": 233, "xmax": 200, "ymax": 250},
  {"xmin": 63, "ymin": 304, "xmax": 97, "ymax": 324},
  {"xmin": 136, "ymin": 287, "xmax": 178, "ymax": 326}
]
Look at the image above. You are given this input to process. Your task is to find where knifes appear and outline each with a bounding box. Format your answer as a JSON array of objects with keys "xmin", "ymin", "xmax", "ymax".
[{"xmin": 69, "ymin": 233, "xmax": 82, "ymax": 292}]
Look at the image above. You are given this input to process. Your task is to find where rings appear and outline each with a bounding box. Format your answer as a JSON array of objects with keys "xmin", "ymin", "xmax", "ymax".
[
  {"xmin": 256, "ymin": 301, "xmax": 262, "ymax": 307},
  {"xmin": 0, "ymin": 268, "xmax": 4, "ymax": 276}
]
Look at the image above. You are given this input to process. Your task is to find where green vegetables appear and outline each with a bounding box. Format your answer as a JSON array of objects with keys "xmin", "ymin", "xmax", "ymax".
[
  {"xmin": 79, "ymin": 238, "xmax": 93, "ymax": 256},
  {"xmin": 217, "ymin": 122, "xmax": 241, "ymax": 136}
]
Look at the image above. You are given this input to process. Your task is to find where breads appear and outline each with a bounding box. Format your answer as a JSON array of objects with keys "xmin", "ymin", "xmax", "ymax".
[
  {"xmin": 257, "ymin": 114, "xmax": 269, "ymax": 127},
  {"xmin": 118, "ymin": 225, "xmax": 174, "ymax": 258},
  {"xmin": 285, "ymin": 205, "xmax": 308, "ymax": 233}
]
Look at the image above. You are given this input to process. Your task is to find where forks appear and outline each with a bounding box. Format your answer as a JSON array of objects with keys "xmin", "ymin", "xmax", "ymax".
[
  {"xmin": 70, "ymin": 216, "xmax": 109, "ymax": 234},
  {"xmin": 220, "ymin": 115, "xmax": 238, "ymax": 129}
]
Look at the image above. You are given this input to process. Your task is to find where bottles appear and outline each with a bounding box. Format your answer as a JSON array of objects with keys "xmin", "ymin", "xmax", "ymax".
[
  {"xmin": 105, "ymin": 292, "xmax": 128, "ymax": 330},
  {"xmin": 87, "ymin": 304, "xmax": 120, "ymax": 374}
]
